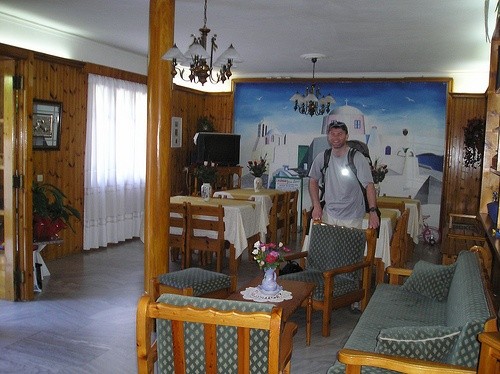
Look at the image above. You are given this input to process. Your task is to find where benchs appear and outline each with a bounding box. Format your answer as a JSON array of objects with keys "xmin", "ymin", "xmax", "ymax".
[{"xmin": 327, "ymin": 213, "xmax": 499, "ymax": 374}]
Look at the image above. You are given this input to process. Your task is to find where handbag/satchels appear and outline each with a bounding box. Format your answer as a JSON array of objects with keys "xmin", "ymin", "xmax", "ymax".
[{"xmin": 279, "ymin": 258, "xmax": 303, "ymax": 275}]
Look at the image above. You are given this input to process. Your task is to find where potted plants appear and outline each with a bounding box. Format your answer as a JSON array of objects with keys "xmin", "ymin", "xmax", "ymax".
[{"xmin": 32, "ymin": 179, "xmax": 82, "ymax": 241}]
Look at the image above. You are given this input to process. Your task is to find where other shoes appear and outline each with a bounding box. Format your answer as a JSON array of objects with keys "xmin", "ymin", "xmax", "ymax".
[{"xmin": 351, "ymin": 302, "xmax": 361, "ymax": 314}]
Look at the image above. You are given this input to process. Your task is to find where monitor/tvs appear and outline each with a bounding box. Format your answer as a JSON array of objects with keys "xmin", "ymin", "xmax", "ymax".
[{"xmin": 196, "ymin": 134, "xmax": 240, "ymax": 167}]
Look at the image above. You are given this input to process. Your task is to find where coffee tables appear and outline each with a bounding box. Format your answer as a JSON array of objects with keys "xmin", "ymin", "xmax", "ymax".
[{"xmin": 224, "ymin": 275, "xmax": 317, "ymax": 347}]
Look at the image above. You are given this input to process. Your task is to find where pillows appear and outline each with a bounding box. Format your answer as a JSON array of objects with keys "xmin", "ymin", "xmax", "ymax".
[
  {"xmin": 399, "ymin": 259, "xmax": 458, "ymax": 303},
  {"xmin": 375, "ymin": 326, "xmax": 462, "ymax": 362}
]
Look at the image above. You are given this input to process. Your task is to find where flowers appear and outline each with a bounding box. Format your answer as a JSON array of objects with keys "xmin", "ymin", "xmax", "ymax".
[
  {"xmin": 246, "ymin": 153, "xmax": 270, "ymax": 178},
  {"xmin": 191, "ymin": 161, "xmax": 219, "ymax": 184},
  {"xmin": 252, "ymin": 240, "xmax": 292, "ymax": 271}
]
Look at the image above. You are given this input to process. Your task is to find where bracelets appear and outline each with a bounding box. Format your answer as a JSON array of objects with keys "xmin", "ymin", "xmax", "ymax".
[{"xmin": 369, "ymin": 207, "xmax": 378, "ymax": 213}]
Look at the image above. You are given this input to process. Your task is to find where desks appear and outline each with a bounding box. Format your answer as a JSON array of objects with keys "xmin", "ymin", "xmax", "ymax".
[
  {"xmin": 375, "ymin": 197, "xmax": 423, "ymax": 245},
  {"xmin": 169, "ymin": 196, "xmax": 267, "ymax": 271},
  {"xmin": 213, "ymin": 188, "xmax": 282, "ymax": 246},
  {"xmin": 300, "ymin": 208, "xmax": 400, "ymax": 273}
]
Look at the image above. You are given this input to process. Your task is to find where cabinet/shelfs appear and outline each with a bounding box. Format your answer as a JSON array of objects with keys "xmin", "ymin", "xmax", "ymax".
[
  {"xmin": 478, "ymin": 15, "xmax": 500, "ymax": 256},
  {"xmin": 188, "ymin": 165, "xmax": 243, "ymax": 196}
]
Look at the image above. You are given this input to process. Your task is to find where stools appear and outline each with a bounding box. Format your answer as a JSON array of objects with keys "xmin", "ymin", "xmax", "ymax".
[{"xmin": 158, "ymin": 267, "xmax": 237, "ymax": 298}]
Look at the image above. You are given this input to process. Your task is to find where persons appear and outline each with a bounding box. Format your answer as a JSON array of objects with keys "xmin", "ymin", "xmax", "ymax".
[{"xmin": 309, "ymin": 122, "xmax": 379, "ymax": 311}]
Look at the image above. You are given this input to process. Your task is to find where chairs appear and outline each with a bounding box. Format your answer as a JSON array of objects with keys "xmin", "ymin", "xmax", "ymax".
[
  {"xmin": 135, "ymin": 292, "xmax": 298, "ymax": 374},
  {"xmin": 170, "ymin": 188, "xmax": 410, "ymax": 337}
]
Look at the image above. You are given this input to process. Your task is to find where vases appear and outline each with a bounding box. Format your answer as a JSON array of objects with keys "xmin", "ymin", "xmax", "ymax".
[
  {"xmin": 254, "ymin": 177, "xmax": 263, "ymax": 192},
  {"xmin": 200, "ymin": 182, "xmax": 213, "ymax": 202},
  {"xmin": 262, "ymin": 266, "xmax": 278, "ymax": 290}
]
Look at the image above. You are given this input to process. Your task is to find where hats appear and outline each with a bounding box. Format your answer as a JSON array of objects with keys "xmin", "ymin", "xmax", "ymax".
[{"xmin": 328, "ymin": 122, "xmax": 348, "ymax": 134}]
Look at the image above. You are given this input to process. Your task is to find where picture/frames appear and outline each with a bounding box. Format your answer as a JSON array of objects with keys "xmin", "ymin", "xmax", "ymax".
[
  {"xmin": 32, "ymin": 98, "xmax": 63, "ymax": 151},
  {"xmin": 171, "ymin": 117, "xmax": 182, "ymax": 148}
]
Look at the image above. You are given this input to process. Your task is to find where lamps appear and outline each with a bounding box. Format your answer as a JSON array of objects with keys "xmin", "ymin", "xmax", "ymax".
[
  {"xmin": 162, "ymin": 0, "xmax": 243, "ymax": 86},
  {"xmin": 289, "ymin": 53, "xmax": 336, "ymax": 117}
]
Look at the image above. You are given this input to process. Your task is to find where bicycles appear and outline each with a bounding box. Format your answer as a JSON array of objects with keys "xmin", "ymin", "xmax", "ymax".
[{"xmin": 421, "ymin": 214, "xmax": 441, "ymax": 246}]
